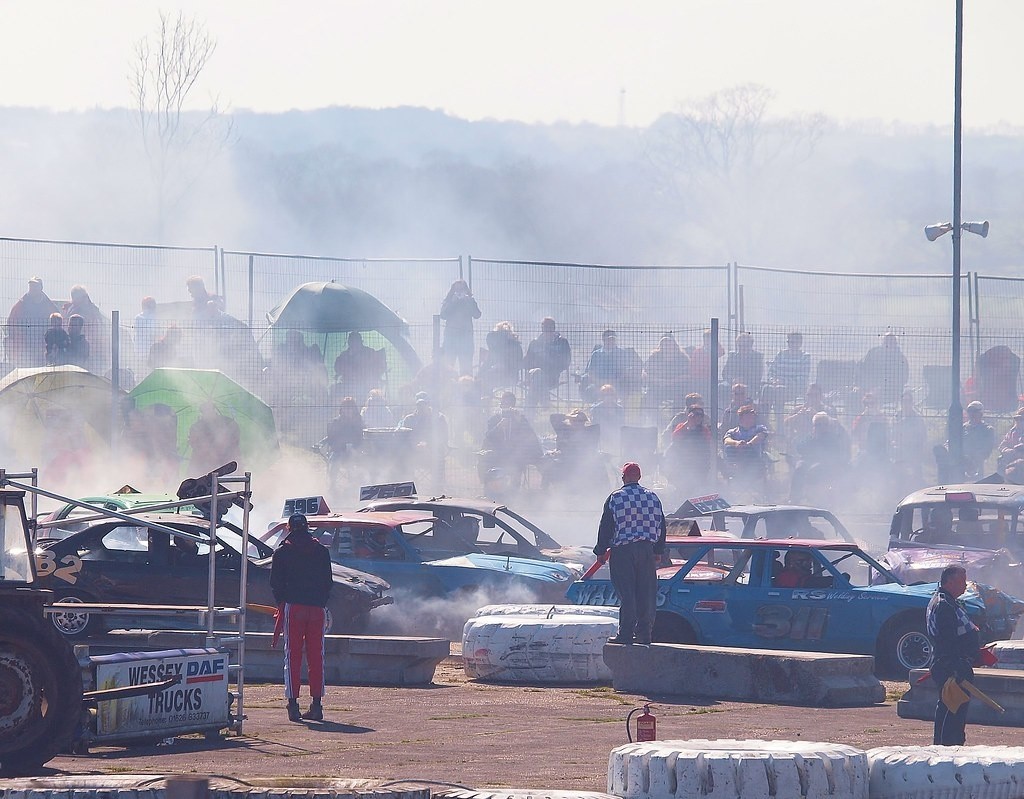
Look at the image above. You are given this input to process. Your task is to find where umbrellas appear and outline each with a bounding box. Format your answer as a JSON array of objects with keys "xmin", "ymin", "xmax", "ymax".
[
  {"xmin": 130, "ymin": 367, "xmax": 278, "ymax": 466},
  {"xmin": 266, "ymin": 278, "xmax": 405, "ymax": 331},
  {"xmin": 0, "ymin": 364, "xmax": 126, "ymax": 469}
]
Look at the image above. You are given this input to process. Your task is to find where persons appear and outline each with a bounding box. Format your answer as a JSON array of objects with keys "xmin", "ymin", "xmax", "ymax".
[
  {"xmin": 719, "ymin": 333, "xmax": 763, "ymax": 410},
  {"xmin": 270, "ymin": 512, "xmax": 332, "ymax": 721},
  {"xmin": 476, "ymin": 317, "xmax": 724, "ymax": 499},
  {"xmin": 144, "ymin": 404, "xmax": 242, "ymax": 481},
  {"xmin": 891, "ymin": 391, "xmax": 925, "ymax": 468},
  {"xmin": 927, "ymin": 566, "xmax": 981, "ymax": 745},
  {"xmin": 172, "ymin": 532, "xmax": 227, "ymax": 567},
  {"xmin": 719, "ymin": 384, "xmax": 888, "ymax": 506},
  {"xmin": 8, "ymin": 276, "xmax": 100, "ymax": 365},
  {"xmin": 269, "ymin": 281, "xmax": 482, "ymax": 495},
  {"xmin": 593, "ymin": 462, "xmax": 666, "ymax": 644},
  {"xmin": 461, "ymin": 517, "xmax": 480, "ymax": 543},
  {"xmin": 761, "ymin": 333, "xmax": 812, "ymax": 420},
  {"xmin": 848, "ymin": 332, "xmax": 909, "ymax": 411},
  {"xmin": 776, "ymin": 549, "xmax": 850, "ymax": 587},
  {"xmin": 933, "ymin": 346, "xmax": 1024, "ymax": 485},
  {"xmin": 138, "ymin": 276, "xmax": 260, "ymax": 379},
  {"xmin": 916, "ymin": 507, "xmax": 958, "ymax": 544}
]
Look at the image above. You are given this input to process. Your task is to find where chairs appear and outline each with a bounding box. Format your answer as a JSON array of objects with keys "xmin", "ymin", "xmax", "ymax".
[
  {"xmin": 770, "ymin": 561, "xmax": 784, "ymax": 578},
  {"xmin": 306, "ymin": 341, "xmax": 968, "ymax": 510}
]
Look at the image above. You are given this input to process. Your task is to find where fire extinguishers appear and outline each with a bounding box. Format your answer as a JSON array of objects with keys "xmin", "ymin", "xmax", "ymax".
[{"xmin": 637, "ymin": 698, "xmax": 659, "ymax": 742}]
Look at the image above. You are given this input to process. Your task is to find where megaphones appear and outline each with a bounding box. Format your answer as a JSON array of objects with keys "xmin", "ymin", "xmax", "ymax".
[
  {"xmin": 924, "ymin": 223, "xmax": 953, "ymax": 242},
  {"xmin": 962, "ymin": 220, "xmax": 989, "ymax": 238}
]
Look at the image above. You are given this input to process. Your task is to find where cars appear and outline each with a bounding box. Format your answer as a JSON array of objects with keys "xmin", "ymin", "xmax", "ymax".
[
  {"xmin": 655, "ymin": 492, "xmax": 883, "ymax": 579},
  {"xmin": 871, "ymin": 483, "xmax": 1024, "ymax": 600},
  {"xmin": 8, "ymin": 482, "xmax": 606, "ymax": 633},
  {"xmin": 564, "ymin": 539, "xmax": 1024, "ymax": 680}
]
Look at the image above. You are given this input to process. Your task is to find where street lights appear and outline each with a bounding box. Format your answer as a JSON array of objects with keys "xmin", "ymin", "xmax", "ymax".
[{"xmin": 926, "ymin": 220, "xmax": 988, "ymax": 481}]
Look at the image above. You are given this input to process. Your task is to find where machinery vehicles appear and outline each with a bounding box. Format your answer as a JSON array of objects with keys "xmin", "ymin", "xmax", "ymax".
[{"xmin": 0, "ymin": 490, "xmax": 82, "ymax": 776}]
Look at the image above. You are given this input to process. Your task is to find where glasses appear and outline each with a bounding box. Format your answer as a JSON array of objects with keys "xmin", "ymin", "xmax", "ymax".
[{"xmin": 694, "ymin": 412, "xmax": 704, "ymax": 417}]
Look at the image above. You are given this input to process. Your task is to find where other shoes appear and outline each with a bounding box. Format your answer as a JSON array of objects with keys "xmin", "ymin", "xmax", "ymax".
[
  {"xmin": 286, "ymin": 703, "xmax": 302, "ymax": 721},
  {"xmin": 634, "ymin": 636, "xmax": 651, "ymax": 644},
  {"xmin": 302, "ymin": 705, "xmax": 323, "ymax": 720},
  {"xmin": 607, "ymin": 636, "xmax": 631, "ymax": 643}
]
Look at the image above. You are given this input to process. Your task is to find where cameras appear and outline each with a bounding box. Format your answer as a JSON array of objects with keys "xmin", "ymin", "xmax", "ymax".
[{"xmin": 456, "ymin": 292, "xmax": 464, "ymax": 300}]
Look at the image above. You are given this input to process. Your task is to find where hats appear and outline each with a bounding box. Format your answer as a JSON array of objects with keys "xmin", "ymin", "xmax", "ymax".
[
  {"xmin": 967, "ymin": 400, "xmax": 984, "ymax": 414},
  {"xmin": 289, "ymin": 513, "xmax": 307, "ymax": 527},
  {"xmin": 620, "ymin": 462, "xmax": 640, "ymax": 478}
]
